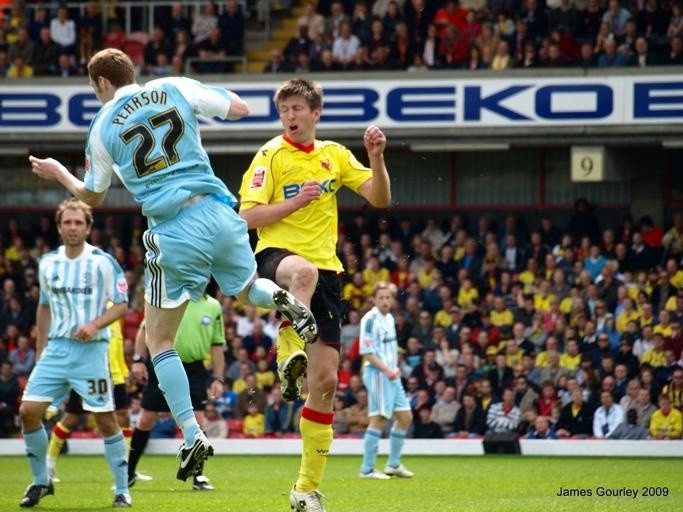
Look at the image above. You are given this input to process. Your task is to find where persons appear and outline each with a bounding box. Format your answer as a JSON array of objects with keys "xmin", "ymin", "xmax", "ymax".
[
  {"xmin": 45, "ymin": 300, "xmax": 153, "ymax": 483},
  {"xmin": 0, "ymin": 211, "xmax": 683, "ymax": 439},
  {"xmin": 112, "ymin": 292, "xmax": 226, "ymax": 492},
  {"xmin": 18, "ymin": 198, "xmax": 130, "ymax": 508},
  {"xmin": 28, "ymin": 48, "xmax": 319, "ymax": 481},
  {"xmin": 0, "ymin": 0, "xmax": 683, "ymax": 77},
  {"xmin": 237, "ymin": 78, "xmax": 391, "ymax": 512},
  {"xmin": 357, "ymin": 281, "xmax": 414, "ymax": 480}
]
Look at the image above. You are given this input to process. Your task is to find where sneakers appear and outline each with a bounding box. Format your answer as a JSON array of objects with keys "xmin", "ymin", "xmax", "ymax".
[
  {"xmin": 136, "ymin": 470, "xmax": 151, "ymax": 483},
  {"xmin": 17, "ymin": 480, "xmax": 55, "ymax": 508},
  {"xmin": 113, "ymin": 492, "xmax": 132, "ymax": 508},
  {"xmin": 190, "ymin": 476, "xmax": 213, "ymax": 492},
  {"xmin": 175, "ymin": 433, "xmax": 214, "ymax": 483},
  {"xmin": 286, "ymin": 485, "xmax": 325, "ymax": 512},
  {"xmin": 385, "ymin": 465, "xmax": 414, "ymax": 479},
  {"xmin": 356, "ymin": 468, "xmax": 389, "ymax": 481},
  {"xmin": 269, "ymin": 290, "xmax": 319, "ymax": 346},
  {"xmin": 106, "ymin": 475, "xmax": 137, "ymax": 491},
  {"xmin": 279, "ymin": 348, "xmax": 309, "ymax": 404}
]
